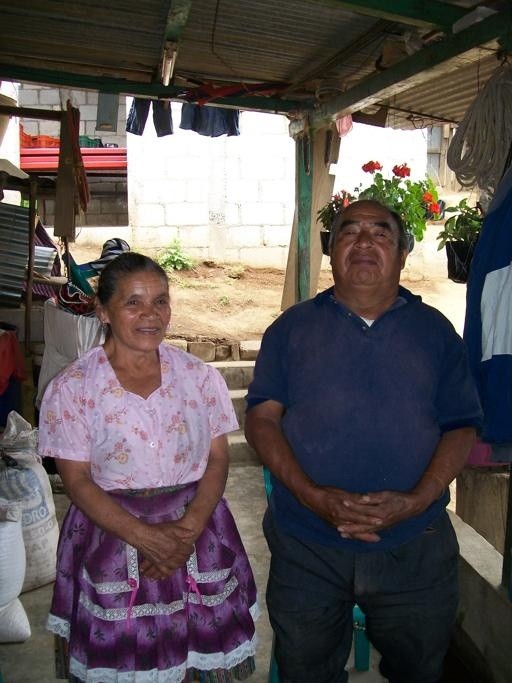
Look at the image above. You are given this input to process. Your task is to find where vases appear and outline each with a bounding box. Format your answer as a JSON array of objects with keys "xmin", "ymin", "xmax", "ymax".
[
  {"xmin": 409, "ymin": 234, "xmax": 417, "ymax": 252},
  {"xmin": 319, "ymin": 229, "xmax": 331, "ymax": 256}
]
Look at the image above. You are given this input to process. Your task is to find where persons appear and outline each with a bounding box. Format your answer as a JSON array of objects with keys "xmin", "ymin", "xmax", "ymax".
[
  {"xmin": 38, "ymin": 253, "xmax": 259, "ymax": 683},
  {"xmin": 245, "ymin": 199, "xmax": 483, "ymax": 683}
]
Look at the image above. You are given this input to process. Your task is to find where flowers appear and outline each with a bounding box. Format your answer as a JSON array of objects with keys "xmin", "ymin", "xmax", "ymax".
[{"xmin": 316, "ymin": 159, "xmax": 445, "ymax": 240}]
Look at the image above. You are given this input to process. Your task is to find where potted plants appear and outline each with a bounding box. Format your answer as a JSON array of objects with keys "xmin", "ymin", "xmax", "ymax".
[{"xmin": 434, "ymin": 197, "xmax": 485, "ymax": 282}]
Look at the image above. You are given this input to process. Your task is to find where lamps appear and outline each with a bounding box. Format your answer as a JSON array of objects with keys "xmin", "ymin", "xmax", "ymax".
[{"xmin": 160, "ymin": 40, "xmax": 179, "ymax": 85}]
